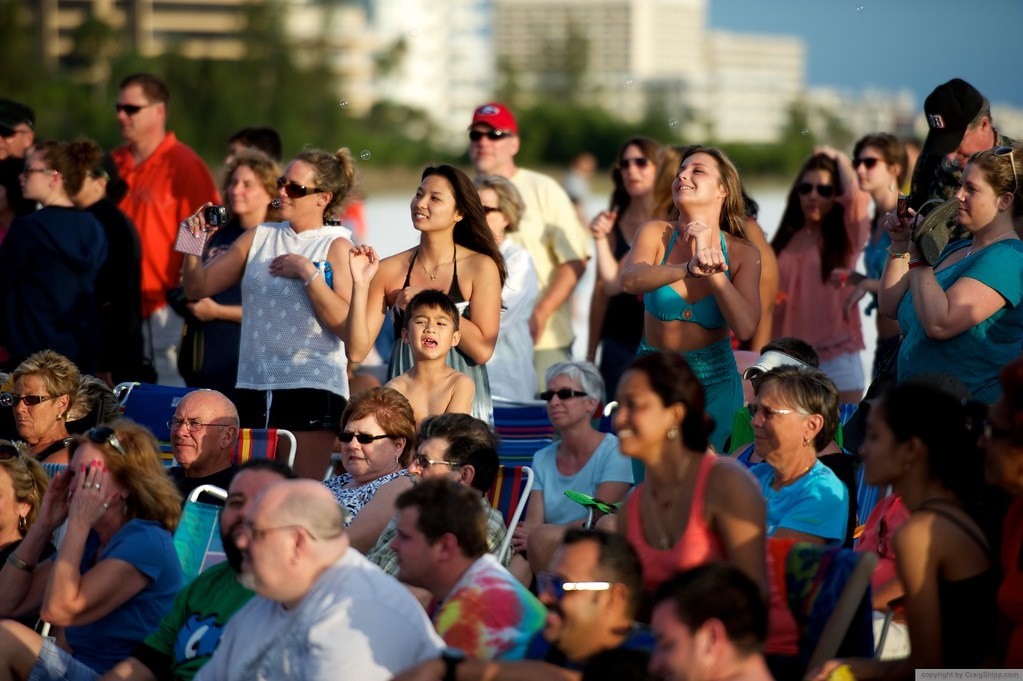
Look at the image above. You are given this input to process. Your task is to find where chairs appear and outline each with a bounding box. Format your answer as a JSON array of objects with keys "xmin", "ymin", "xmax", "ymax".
[{"xmin": 103, "ymin": 381, "xmax": 897, "ymax": 681}]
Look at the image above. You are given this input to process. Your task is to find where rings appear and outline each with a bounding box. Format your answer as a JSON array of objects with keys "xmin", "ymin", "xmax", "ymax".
[
  {"xmin": 84, "ymin": 481, "xmax": 91, "ymax": 488},
  {"xmin": 276, "ymin": 265, "xmax": 281, "ymax": 269},
  {"xmin": 103, "ymin": 502, "xmax": 109, "ymax": 509},
  {"xmin": 93, "ymin": 482, "xmax": 101, "ymax": 490}
]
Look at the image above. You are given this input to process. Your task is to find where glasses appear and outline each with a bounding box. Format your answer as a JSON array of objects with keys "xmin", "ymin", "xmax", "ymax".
[
  {"xmin": 88, "ymin": 427, "xmax": 127, "ymax": 459},
  {"xmin": 468, "ymin": 131, "xmax": 512, "ymax": 141},
  {"xmin": 981, "ymin": 424, "xmax": 1023, "ymax": 447},
  {"xmin": 338, "ymin": 432, "xmax": 398, "ymax": 443},
  {"xmin": 23, "ymin": 164, "xmax": 59, "ymax": 179},
  {"xmin": 748, "ymin": 403, "xmax": 811, "ymax": 419},
  {"xmin": 853, "ymin": 158, "xmax": 886, "ymax": 170},
  {"xmin": 242, "ymin": 524, "xmax": 317, "ymax": 542},
  {"xmin": 536, "ymin": 571, "xmax": 614, "ymax": 600},
  {"xmin": 483, "ymin": 206, "xmax": 501, "ymax": 214},
  {"xmin": 798, "ymin": 183, "xmax": 833, "ymax": 198},
  {"xmin": 6, "ymin": 393, "xmax": 72, "ymax": 407},
  {"xmin": 413, "ymin": 453, "xmax": 459, "ymax": 468},
  {"xmin": 277, "ymin": 177, "xmax": 327, "ymax": 198},
  {"xmin": 0, "ymin": 444, "xmax": 34, "ymax": 487},
  {"xmin": 540, "ymin": 389, "xmax": 587, "ymax": 400},
  {"xmin": 167, "ymin": 418, "xmax": 229, "ymax": 431},
  {"xmin": 991, "ymin": 145, "xmax": 1018, "ymax": 195},
  {"xmin": 620, "ymin": 158, "xmax": 653, "ymax": 167},
  {"xmin": 115, "ymin": 103, "xmax": 154, "ymax": 115},
  {"xmin": 0, "ymin": 125, "xmax": 27, "ymax": 137}
]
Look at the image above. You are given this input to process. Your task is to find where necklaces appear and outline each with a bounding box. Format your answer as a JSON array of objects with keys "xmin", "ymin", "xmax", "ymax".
[
  {"xmin": 426, "ymin": 267, "xmax": 439, "ymax": 280},
  {"xmin": 646, "ymin": 452, "xmax": 693, "ymax": 551}
]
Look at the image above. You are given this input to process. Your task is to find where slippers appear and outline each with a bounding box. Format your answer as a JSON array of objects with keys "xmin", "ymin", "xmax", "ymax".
[{"xmin": 911, "ymin": 197, "xmax": 961, "ymax": 267}]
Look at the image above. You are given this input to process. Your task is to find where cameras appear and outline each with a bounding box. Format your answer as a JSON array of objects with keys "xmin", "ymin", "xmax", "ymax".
[
  {"xmin": 897, "ymin": 195, "xmax": 914, "ymax": 219},
  {"xmin": 204, "ymin": 206, "xmax": 229, "ymax": 227}
]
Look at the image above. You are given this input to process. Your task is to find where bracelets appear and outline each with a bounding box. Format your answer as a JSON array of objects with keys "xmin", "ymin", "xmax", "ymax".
[
  {"xmin": 6, "ymin": 551, "xmax": 37, "ymax": 573},
  {"xmin": 907, "ymin": 257, "xmax": 919, "ymax": 264},
  {"xmin": 908, "ymin": 261, "xmax": 932, "ymax": 269},
  {"xmin": 687, "ymin": 260, "xmax": 701, "ymax": 278},
  {"xmin": 886, "ymin": 245, "xmax": 911, "ymax": 259},
  {"xmin": 303, "ymin": 269, "xmax": 320, "ymax": 287}
]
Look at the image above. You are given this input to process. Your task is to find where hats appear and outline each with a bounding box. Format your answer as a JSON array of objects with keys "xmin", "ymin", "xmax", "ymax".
[
  {"xmin": 743, "ymin": 350, "xmax": 811, "ymax": 380},
  {"xmin": 468, "ymin": 103, "xmax": 519, "ymax": 134},
  {"xmin": 924, "ymin": 79, "xmax": 983, "ymax": 155},
  {"xmin": 0, "ymin": 98, "xmax": 35, "ymax": 129}
]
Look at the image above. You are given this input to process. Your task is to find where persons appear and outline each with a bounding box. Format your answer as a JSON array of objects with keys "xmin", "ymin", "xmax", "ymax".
[
  {"xmin": 192, "ymin": 477, "xmax": 448, "ymax": 681},
  {"xmin": 0, "ymin": 72, "xmax": 1023, "ymax": 681},
  {"xmin": 618, "ymin": 143, "xmax": 762, "ymax": 488},
  {"xmin": 345, "ymin": 164, "xmax": 510, "ymax": 435},
  {"xmin": 0, "ymin": 417, "xmax": 185, "ymax": 681},
  {"xmin": 321, "ymin": 386, "xmax": 417, "ymax": 555},
  {"xmin": 612, "ymin": 349, "xmax": 770, "ymax": 608},
  {"xmin": 166, "ymin": 389, "xmax": 241, "ymax": 511},
  {"xmin": 182, "ymin": 142, "xmax": 355, "ymax": 481}
]
